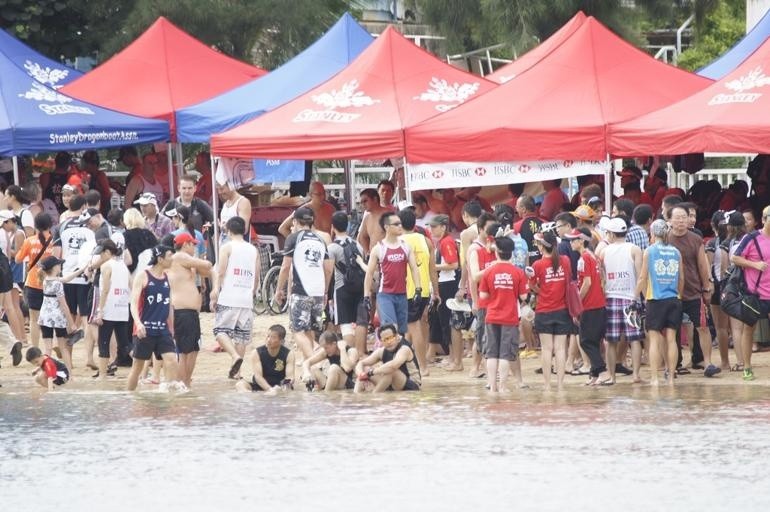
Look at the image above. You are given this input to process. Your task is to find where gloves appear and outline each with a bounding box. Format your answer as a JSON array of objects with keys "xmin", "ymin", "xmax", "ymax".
[
  {"xmin": 412, "ymin": 287, "xmax": 422, "ymax": 303},
  {"xmin": 363, "ymin": 296, "xmax": 371, "ymax": 311}
]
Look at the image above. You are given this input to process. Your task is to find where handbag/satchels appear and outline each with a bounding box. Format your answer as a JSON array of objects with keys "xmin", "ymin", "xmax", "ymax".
[{"xmin": 721, "ymin": 266, "xmax": 761, "ymax": 326}]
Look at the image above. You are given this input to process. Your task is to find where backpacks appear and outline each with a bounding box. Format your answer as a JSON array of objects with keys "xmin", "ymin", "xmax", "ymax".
[{"xmin": 335, "ymin": 240, "xmax": 364, "ymax": 293}]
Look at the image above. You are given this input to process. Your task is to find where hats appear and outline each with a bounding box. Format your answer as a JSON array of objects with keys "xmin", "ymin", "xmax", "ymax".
[
  {"xmin": 0, "ymin": 209, "xmax": 15, "ymax": 228},
  {"xmin": 534, "ymin": 205, "xmax": 627, "ymax": 247},
  {"xmin": 719, "ymin": 210, "xmax": 745, "ymax": 226},
  {"xmin": 61, "ymin": 184, "xmax": 75, "ymax": 193},
  {"xmin": 165, "ymin": 208, "xmax": 184, "ymax": 219},
  {"xmin": 294, "ymin": 207, "xmax": 314, "ymax": 222},
  {"xmin": 79, "ymin": 208, "xmax": 98, "ymax": 222},
  {"xmin": 425, "ymin": 214, "xmax": 449, "ymax": 226},
  {"xmin": 148, "ymin": 232, "xmax": 199, "ymax": 265},
  {"xmin": 133, "ymin": 192, "xmax": 157, "ymax": 205},
  {"xmin": 42, "ymin": 256, "xmax": 65, "ymax": 270}
]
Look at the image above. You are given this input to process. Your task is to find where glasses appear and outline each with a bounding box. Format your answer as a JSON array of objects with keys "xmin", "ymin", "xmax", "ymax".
[{"xmin": 390, "ymin": 223, "xmax": 400, "ymax": 226}]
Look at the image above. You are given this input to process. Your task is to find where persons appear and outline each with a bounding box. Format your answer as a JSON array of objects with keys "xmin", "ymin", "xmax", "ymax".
[{"xmin": 1, "ymin": 148, "xmax": 770, "ymax": 395}]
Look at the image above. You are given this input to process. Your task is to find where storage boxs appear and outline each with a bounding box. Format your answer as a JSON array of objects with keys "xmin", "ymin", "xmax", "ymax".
[{"xmin": 239, "ymin": 184, "xmax": 275, "ymax": 207}]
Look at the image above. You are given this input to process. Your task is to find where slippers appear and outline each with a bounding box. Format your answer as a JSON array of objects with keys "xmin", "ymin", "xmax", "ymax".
[
  {"xmin": 663, "ymin": 363, "xmax": 754, "ymax": 381},
  {"xmin": 229, "ymin": 359, "xmax": 242, "ymax": 379},
  {"xmin": 11, "ymin": 342, "xmax": 22, "ymax": 365},
  {"xmin": 535, "ymin": 365, "xmax": 648, "ymax": 386},
  {"xmin": 68, "ymin": 329, "xmax": 83, "ymax": 346},
  {"xmin": 93, "ymin": 363, "xmax": 160, "ymax": 384}
]
